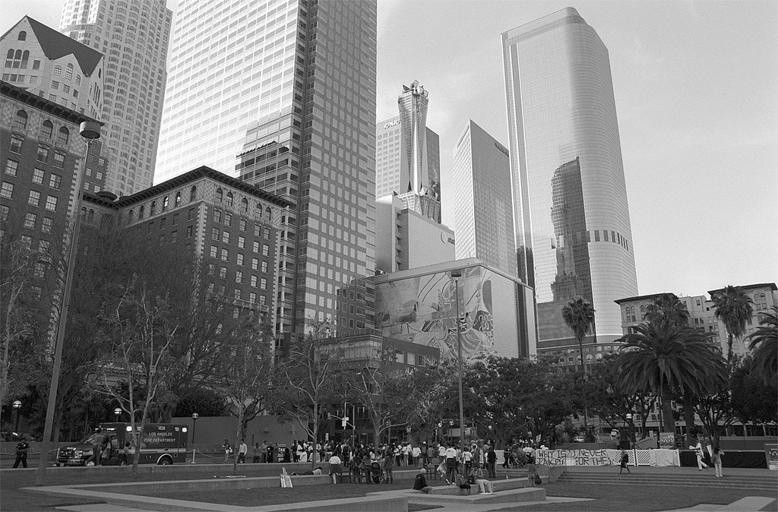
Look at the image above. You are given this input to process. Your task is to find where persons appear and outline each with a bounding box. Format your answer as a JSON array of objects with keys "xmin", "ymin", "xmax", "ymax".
[
  {"xmin": 618, "ymin": 451, "xmax": 630, "ymax": 474},
  {"xmin": 12, "ymin": 438, "xmax": 29, "ymax": 468},
  {"xmin": 692, "ymin": 443, "xmax": 708, "ymax": 471},
  {"xmin": 711, "ymin": 447, "xmax": 725, "ymax": 478}
]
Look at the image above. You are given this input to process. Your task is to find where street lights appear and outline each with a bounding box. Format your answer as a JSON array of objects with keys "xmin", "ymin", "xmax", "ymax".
[
  {"xmin": 191, "ymin": 411, "xmax": 198, "ymax": 443},
  {"xmin": 626, "ymin": 413, "xmax": 634, "ymax": 450},
  {"xmin": 12, "ymin": 399, "xmax": 23, "ymax": 432},
  {"xmin": 114, "ymin": 406, "xmax": 122, "ymax": 422},
  {"xmin": 449, "ymin": 270, "xmax": 466, "ymax": 463}
]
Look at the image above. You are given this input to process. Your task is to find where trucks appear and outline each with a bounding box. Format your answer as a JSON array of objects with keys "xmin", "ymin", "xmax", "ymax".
[{"xmin": 57, "ymin": 420, "xmax": 189, "ymax": 465}]
